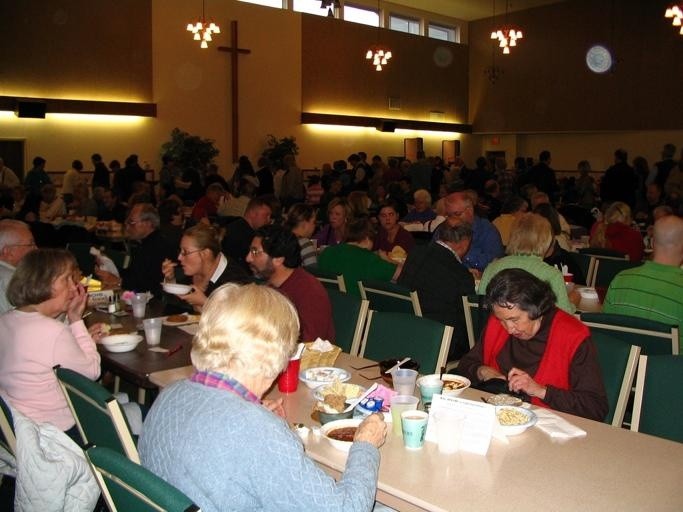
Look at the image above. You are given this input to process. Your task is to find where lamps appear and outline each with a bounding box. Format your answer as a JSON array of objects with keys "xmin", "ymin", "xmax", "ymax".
[
  {"xmin": 365, "ymin": 30, "xmax": 393, "ymax": 71},
  {"xmin": 184, "ymin": 0, "xmax": 222, "ymax": 50},
  {"xmin": 490, "ymin": 0, "xmax": 527, "ymax": 56},
  {"xmin": 662, "ymin": 0, "xmax": 683, "ymax": 40}
]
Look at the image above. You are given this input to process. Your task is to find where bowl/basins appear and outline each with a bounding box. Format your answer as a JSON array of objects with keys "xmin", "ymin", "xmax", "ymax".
[
  {"xmin": 577, "ymin": 287, "xmax": 597, "ymax": 301},
  {"xmin": 318, "ymin": 405, "xmax": 353, "ymax": 424},
  {"xmin": 159, "ymin": 282, "xmax": 193, "ymax": 295},
  {"xmin": 378, "ymin": 359, "xmax": 420, "ymax": 386},
  {"xmin": 493, "ymin": 405, "xmax": 537, "ymax": 435},
  {"xmin": 564, "ymin": 280, "xmax": 574, "ymax": 295},
  {"xmin": 96, "ymin": 333, "xmax": 142, "ymax": 353},
  {"xmin": 298, "ymin": 367, "xmax": 351, "ymax": 389},
  {"xmin": 317, "ymin": 419, "xmax": 362, "ymax": 453},
  {"xmin": 417, "ymin": 374, "xmax": 470, "ymax": 397},
  {"xmin": 310, "ymin": 384, "xmax": 366, "ymax": 402}
]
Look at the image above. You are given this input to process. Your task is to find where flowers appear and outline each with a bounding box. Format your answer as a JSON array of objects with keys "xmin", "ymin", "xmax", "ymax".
[
  {"xmin": 164, "ymin": 126, "xmax": 218, "ymax": 174},
  {"xmin": 260, "ymin": 133, "xmax": 302, "ymax": 167}
]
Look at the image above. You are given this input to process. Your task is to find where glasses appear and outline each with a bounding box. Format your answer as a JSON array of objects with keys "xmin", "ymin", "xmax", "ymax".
[
  {"xmin": 125, "ymin": 219, "xmax": 140, "ymax": 225},
  {"xmin": 443, "ymin": 206, "xmax": 468, "ymax": 219},
  {"xmin": 180, "ymin": 249, "xmax": 202, "ymax": 256},
  {"xmin": 250, "ymin": 247, "xmax": 265, "ymax": 257},
  {"xmin": 8, "ymin": 241, "xmax": 36, "ymax": 251}
]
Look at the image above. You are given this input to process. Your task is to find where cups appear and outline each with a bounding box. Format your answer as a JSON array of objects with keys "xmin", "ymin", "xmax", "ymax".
[
  {"xmin": 142, "ymin": 318, "xmax": 163, "ymax": 345},
  {"xmin": 399, "ymin": 409, "xmax": 427, "ymax": 450},
  {"xmin": 129, "ymin": 293, "xmax": 146, "ymax": 317},
  {"xmin": 388, "ymin": 395, "xmax": 417, "ymax": 436},
  {"xmin": 390, "ymin": 368, "xmax": 418, "ymax": 395},
  {"xmin": 277, "ymin": 357, "xmax": 300, "ymax": 394},
  {"xmin": 420, "ymin": 379, "xmax": 443, "ymax": 409}
]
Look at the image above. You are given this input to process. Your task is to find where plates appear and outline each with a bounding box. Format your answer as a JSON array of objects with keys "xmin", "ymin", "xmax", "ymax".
[{"xmin": 160, "ymin": 313, "xmax": 195, "ymax": 327}]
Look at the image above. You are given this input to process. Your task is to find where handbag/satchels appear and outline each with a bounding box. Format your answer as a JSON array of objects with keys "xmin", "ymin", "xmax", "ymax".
[{"xmin": 482, "ymin": 377, "xmax": 529, "ymax": 402}]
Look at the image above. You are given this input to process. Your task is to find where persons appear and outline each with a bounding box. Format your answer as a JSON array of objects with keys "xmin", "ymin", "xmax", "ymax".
[
  {"xmin": 1, "ymin": 144, "xmax": 683, "ymax": 323},
  {"xmin": 134, "ymin": 285, "xmax": 386, "ymax": 511},
  {"xmin": 458, "ymin": 268, "xmax": 609, "ymax": 422},
  {"xmin": 162, "ymin": 221, "xmax": 248, "ymax": 314},
  {"xmin": 245, "ymin": 225, "xmax": 334, "ymax": 345},
  {"xmin": 0, "ymin": 249, "xmax": 141, "ymax": 475},
  {"xmin": 0, "ymin": 219, "xmax": 41, "ymax": 312}
]
